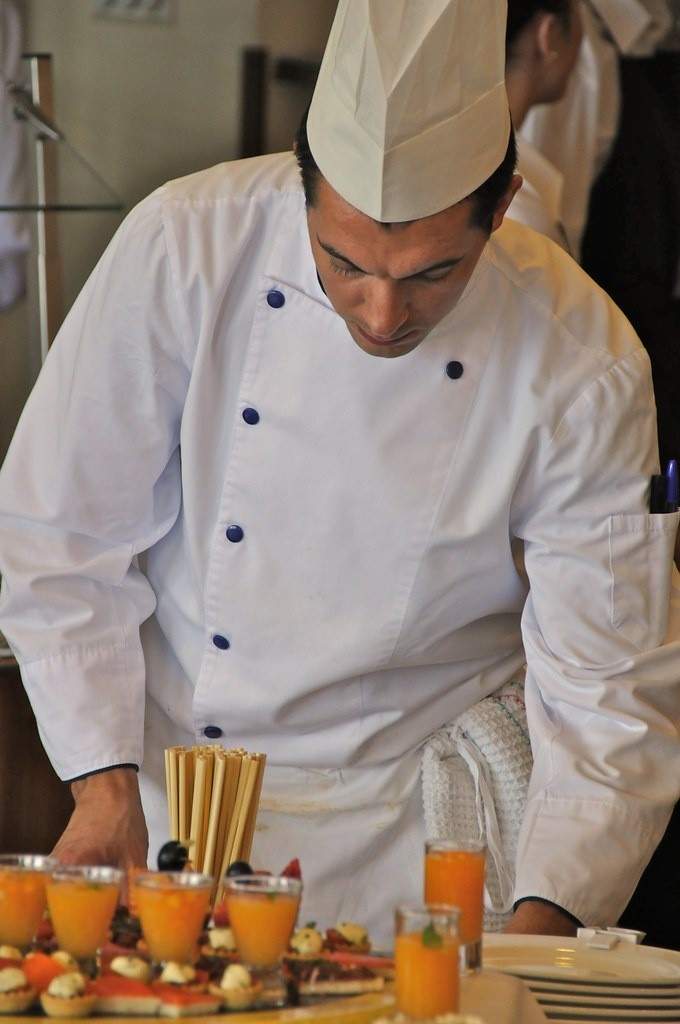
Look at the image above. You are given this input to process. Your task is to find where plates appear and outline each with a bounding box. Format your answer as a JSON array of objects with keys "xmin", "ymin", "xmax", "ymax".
[
  {"xmin": 478, "ymin": 930, "xmax": 680, "ymax": 1024},
  {"xmin": 0, "ymin": 963, "xmax": 396, "ymax": 1023}
]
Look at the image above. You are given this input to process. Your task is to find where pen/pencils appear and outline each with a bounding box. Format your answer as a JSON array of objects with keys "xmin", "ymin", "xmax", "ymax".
[{"xmin": 667, "ymin": 459, "xmax": 678, "ymax": 512}]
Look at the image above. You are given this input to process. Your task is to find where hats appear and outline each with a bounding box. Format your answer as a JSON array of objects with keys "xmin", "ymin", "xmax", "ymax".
[{"xmin": 306, "ymin": 1, "xmax": 511, "ymax": 224}]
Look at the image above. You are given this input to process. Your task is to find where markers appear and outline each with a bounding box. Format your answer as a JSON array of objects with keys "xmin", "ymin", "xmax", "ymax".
[{"xmin": 650, "ymin": 475, "xmax": 670, "ymax": 514}]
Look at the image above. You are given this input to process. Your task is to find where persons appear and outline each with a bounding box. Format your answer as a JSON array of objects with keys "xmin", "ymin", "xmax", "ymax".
[{"xmin": 0, "ymin": 0, "xmax": 680, "ymax": 936}]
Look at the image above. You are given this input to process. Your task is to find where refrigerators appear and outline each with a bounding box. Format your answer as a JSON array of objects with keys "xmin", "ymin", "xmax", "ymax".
[{"xmin": 1, "ymin": 0, "xmax": 336, "ymax": 665}]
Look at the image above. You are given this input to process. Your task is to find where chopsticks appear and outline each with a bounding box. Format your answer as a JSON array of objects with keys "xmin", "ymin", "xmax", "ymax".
[{"xmin": 165, "ymin": 745, "xmax": 267, "ymax": 914}]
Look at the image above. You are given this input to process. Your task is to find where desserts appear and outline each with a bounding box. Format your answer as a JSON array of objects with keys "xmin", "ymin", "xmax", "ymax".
[{"xmin": 0, "ymin": 922, "xmax": 400, "ymax": 1018}]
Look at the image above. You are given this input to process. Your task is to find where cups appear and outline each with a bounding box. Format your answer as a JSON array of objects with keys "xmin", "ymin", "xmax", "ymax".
[
  {"xmin": 0, "ymin": 854, "xmax": 62, "ymax": 952},
  {"xmin": 136, "ymin": 872, "xmax": 216, "ymax": 969},
  {"xmin": 391, "ymin": 903, "xmax": 461, "ymax": 1024},
  {"xmin": 425, "ymin": 837, "xmax": 488, "ymax": 976},
  {"xmin": 221, "ymin": 874, "xmax": 304, "ymax": 984},
  {"xmin": 44, "ymin": 865, "xmax": 126, "ymax": 974}
]
